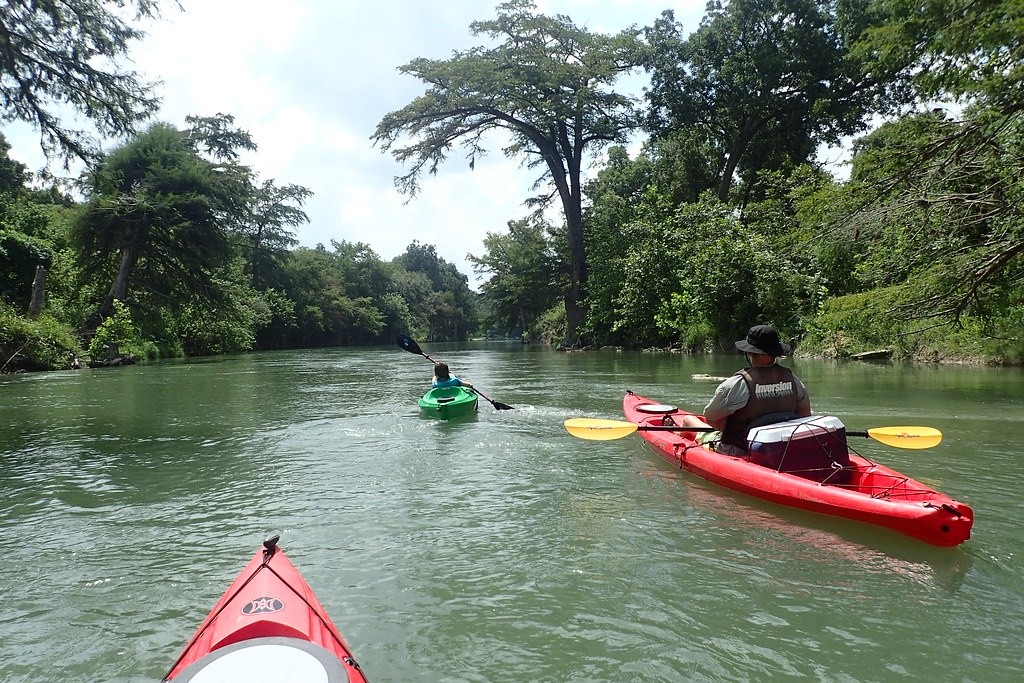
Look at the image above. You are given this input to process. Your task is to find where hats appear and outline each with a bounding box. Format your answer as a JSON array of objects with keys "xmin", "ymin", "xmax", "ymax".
[{"xmin": 735, "ymin": 325, "xmax": 791, "ymax": 357}]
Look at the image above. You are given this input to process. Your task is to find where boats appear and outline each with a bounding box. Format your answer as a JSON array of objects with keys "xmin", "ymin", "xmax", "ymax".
[
  {"xmin": 849, "ymin": 350, "xmax": 894, "ymax": 359},
  {"xmin": 161, "ymin": 535, "xmax": 368, "ymax": 683},
  {"xmin": 622, "ymin": 388, "xmax": 973, "ymax": 548},
  {"xmin": 418, "ymin": 386, "xmax": 478, "ymax": 422}
]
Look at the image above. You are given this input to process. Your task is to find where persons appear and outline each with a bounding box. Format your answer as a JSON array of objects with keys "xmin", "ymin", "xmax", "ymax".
[
  {"xmin": 432, "ymin": 362, "xmax": 473, "ymax": 390},
  {"xmin": 662, "ymin": 326, "xmax": 812, "ymax": 459}
]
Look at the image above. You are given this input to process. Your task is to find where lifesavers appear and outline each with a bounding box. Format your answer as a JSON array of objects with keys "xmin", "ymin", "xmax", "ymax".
[{"xmin": 436, "ymin": 395, "xmax": 456, "ymax": 402}]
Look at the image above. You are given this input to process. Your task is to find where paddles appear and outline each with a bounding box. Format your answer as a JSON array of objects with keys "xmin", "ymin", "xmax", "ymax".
[
  {"xmin": 396, "ymin": 333, "xmax": 516, "ymax": 410},
  {"xmin": 563, "ymin": 417, "xmax": 950, "ymax": 450}
]
